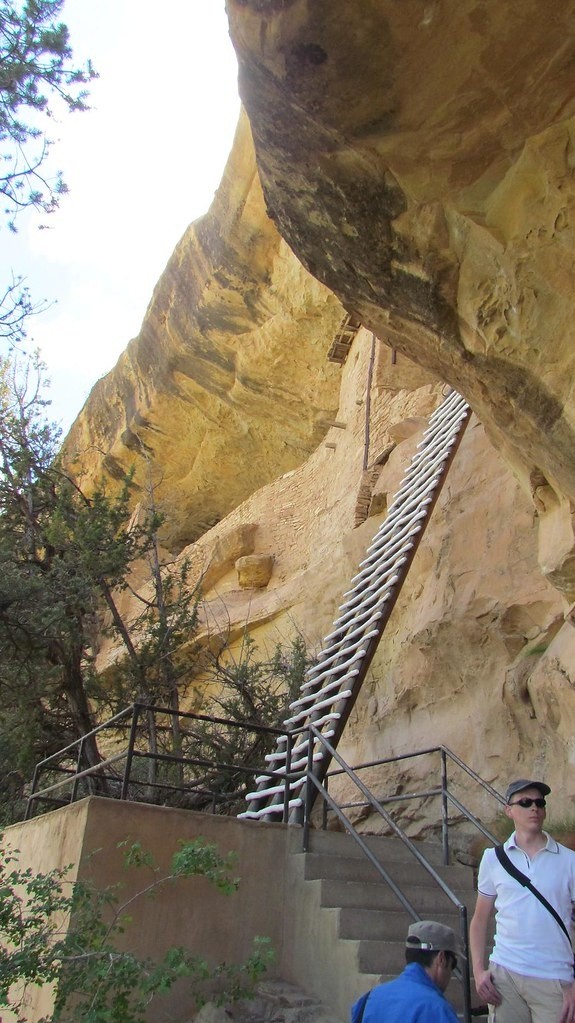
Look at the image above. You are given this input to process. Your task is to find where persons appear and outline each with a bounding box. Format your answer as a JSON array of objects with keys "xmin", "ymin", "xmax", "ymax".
[
  {"xmin": 351, "ymin": 920, "xmax": 467, "ymax": 1023},
  {"xmin": 470, "ymin": 779, "xmax": 575, "ymax": 1023}
]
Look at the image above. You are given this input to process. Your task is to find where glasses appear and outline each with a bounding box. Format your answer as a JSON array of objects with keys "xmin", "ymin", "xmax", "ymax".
[
  {"xmin": 446, "ymin": 952, "xmax": 457, "ymax": 970},
  {"xmin": 509, "ymin": 797, "xmax": 547, "ymax": 808}
]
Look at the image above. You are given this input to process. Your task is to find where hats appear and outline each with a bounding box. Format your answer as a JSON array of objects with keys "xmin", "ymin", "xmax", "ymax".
[
  {"xmin": 406, "ymin": 920, "xmax": 467, "ymax": 961},
  {"xmin": 506, "ymin": 779, "xmax": 551, "ymax": 804}
]
